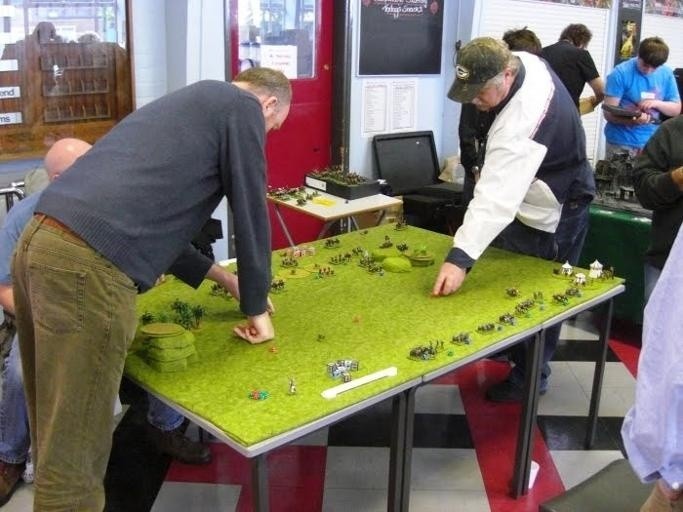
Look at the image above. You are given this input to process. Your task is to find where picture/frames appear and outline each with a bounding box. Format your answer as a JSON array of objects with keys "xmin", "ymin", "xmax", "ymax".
[{"xmin": 355, "ymin": 1, "xmax": 446, "ymax": 79}]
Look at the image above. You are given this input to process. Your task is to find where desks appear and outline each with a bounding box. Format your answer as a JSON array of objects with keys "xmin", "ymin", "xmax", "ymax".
[
  {"xmin": 267, "ymin": 186, "xmax": 403, "ymax": 247},
  {"xmin": 125, "ymin": 221, "xmax": 626, "ymax": 511}
]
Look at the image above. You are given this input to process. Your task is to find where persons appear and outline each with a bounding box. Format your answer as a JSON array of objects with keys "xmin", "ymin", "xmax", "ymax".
[
  {"xmin": 11, "ymin": 67, "xmax": 293, "ymax": 511},
  {"xmin": 432, "ymin": 37, "xmax": 597, "ymax": 402},
  {"xmin": 621, "ymin": 220, "xmax": 682, "ymax": 512},
  {"xmin": 458, "ymin": 27, "xmax": 541, "ymax": 209},
  {"xmin": 602, "ymin": 37, "xmax": 682, "ymax": 162},
  {"xmin": 0, "ymin": 138, "xmax": 213, "ymax": 506},
  {"xmin": 633, "ymin": 114, "xmax": 683, "ymax": 307},
  {"xmin": 5, "ymin": 15, "xmax": 129, "ymax": 128},
  {"xmin": 541, "ymin": 23, "xmax": 604, "ymax": 116}
]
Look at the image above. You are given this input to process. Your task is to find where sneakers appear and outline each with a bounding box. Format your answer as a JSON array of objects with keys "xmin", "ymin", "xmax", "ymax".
[
  {"xmin": 145, "ymin": 428, "xmax": 214, "ymax": 466},
  {"xmin": 0, "ymin": 458, "xmax": 31, "ymax": 509}
]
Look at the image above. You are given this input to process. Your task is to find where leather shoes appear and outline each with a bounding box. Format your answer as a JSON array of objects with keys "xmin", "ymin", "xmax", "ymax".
[{"xmin": 488, "ymin": 375, "xmax": 549, "ymax": 403}]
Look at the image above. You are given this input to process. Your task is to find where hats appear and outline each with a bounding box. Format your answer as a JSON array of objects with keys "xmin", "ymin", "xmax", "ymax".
[{"xmin": 446, "ymin": 36, "xmax": 510, "ymax": 104}]
